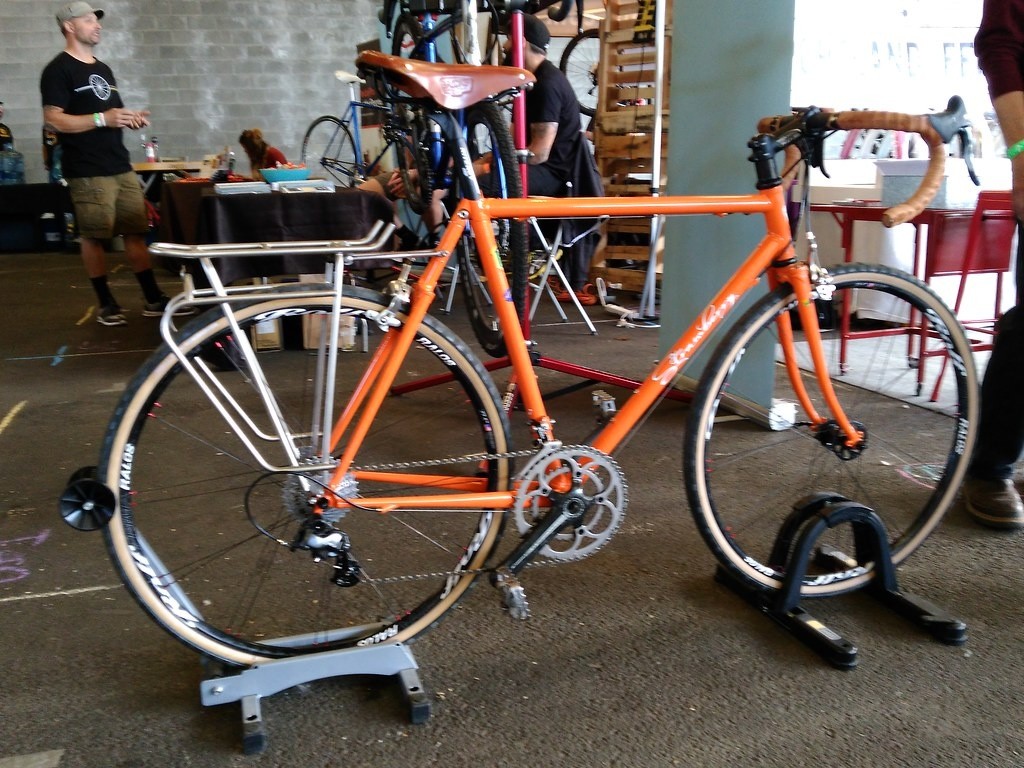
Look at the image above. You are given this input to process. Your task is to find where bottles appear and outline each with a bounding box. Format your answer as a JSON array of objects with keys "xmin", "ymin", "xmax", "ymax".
[
  {"xmin": 151, "ymin": 135, "xmax": 160, "ymax": 162},
  {"xmin": 229, "ymin": 152, "xmax": 236, "ymax": 176}
]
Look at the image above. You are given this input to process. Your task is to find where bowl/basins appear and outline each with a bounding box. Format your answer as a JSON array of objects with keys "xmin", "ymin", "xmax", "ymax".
[{"xmin": 258, "ymin": 168, "xmax": 311, "ymax": 187}]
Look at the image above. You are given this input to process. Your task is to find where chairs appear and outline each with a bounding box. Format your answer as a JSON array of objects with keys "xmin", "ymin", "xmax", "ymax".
[
  {"xmin": 930, "ymin": 190, "xmax": 1017, "ymax": 400},
  {"xmin": 442, "ymin": 131, "xmax": 610, "ymax": 336}
]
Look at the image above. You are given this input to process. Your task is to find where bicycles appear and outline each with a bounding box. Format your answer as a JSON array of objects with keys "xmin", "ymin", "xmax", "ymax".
[
  {"xmin": 97, "ymin": 47, "xmax": 984, "ymax": 673},
  {"xmin": 301, "ymin": 68, "xmax": 421, "ymax": 189},
  {"xmin": 558, "ymin": 30, "xmax": 624, "ymax": 116},
  {"xmin": 378, "ymin": 0, "xmax": 572, "ymax": 359}
]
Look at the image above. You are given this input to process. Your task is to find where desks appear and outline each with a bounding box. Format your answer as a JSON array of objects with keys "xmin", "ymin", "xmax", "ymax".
[
  {"xmin": 202, "ymin": 187, "xmax": 395, "ymax": 286},
  {"xmin": 806, "ymin": 203, "xmax": 1016, "ymax": 395},
  {"xmin": 158, "ymin": 176, "xmax": 247, "ymax": 245},
  {"xmin": 130, "ymin": 162, "xmax": 203, "ymax": 245}
]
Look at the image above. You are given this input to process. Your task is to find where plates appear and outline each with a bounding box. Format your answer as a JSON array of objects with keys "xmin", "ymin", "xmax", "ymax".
[{"xmin": 833, "ymin": 199, "xmax": 869, "ymax": 207}]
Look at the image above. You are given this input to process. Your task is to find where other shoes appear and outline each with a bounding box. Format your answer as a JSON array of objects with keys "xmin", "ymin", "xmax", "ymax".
[
  {"xmin": 962, "ymin": 475, "xmax": 1024, "ymax": 525},
  {"xmin": 141, "ymin": 296, "xmax": 194, "ymax": 316},
  {"xmin": 95, "ymin": 311, "xmax": 127, "ymax": 326}
]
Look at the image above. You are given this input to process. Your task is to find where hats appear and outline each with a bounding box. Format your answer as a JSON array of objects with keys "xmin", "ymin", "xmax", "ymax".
[
  {"xmin": 56, "ymin": 1, "xmax": 106, "ymax": 28},
  {"xmin": 499, "ymin": 14, "xmax": 551, "ymax": 54}
]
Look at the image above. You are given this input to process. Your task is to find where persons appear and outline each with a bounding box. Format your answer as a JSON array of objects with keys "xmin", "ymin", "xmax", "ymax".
[
  {"xmin": 41, "ymin": 122, "xmax": 57, "ymax": 171},
  {"xmin": 955, "ymin": 0, "xmax": 1024, "ymax": 532},
  {"xmin": 0, "ymin": 100, "xmax": 14, "ymax": 152},
  {"xmin": 238, "ymin": 128, "xmax": 287, "ymax": 183},
  {"xmin": 39, "ymin": 1, "xmax": 197, "ymax": 327},
  {"xmin": 356, "ymin": 14, "xmax": 582, "ymax": 261}
]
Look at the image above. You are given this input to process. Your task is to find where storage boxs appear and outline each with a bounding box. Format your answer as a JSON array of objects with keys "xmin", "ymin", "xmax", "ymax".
[{"xmin": 297, "ymin": 272, "xmax": 357, "ymax": 349}]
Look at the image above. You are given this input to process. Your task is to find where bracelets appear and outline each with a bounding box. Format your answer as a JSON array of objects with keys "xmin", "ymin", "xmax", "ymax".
[
  {"xmin": 94, "ymin": 113, "xmax": 102, "ymax": 128},
  {"xmin": 99, "ymin": 113, "xmax": 106, "ymax": 126},
  {"xmin": 1005, "ymin": 140, "xmax": 1023, "ymax": 160}
]
